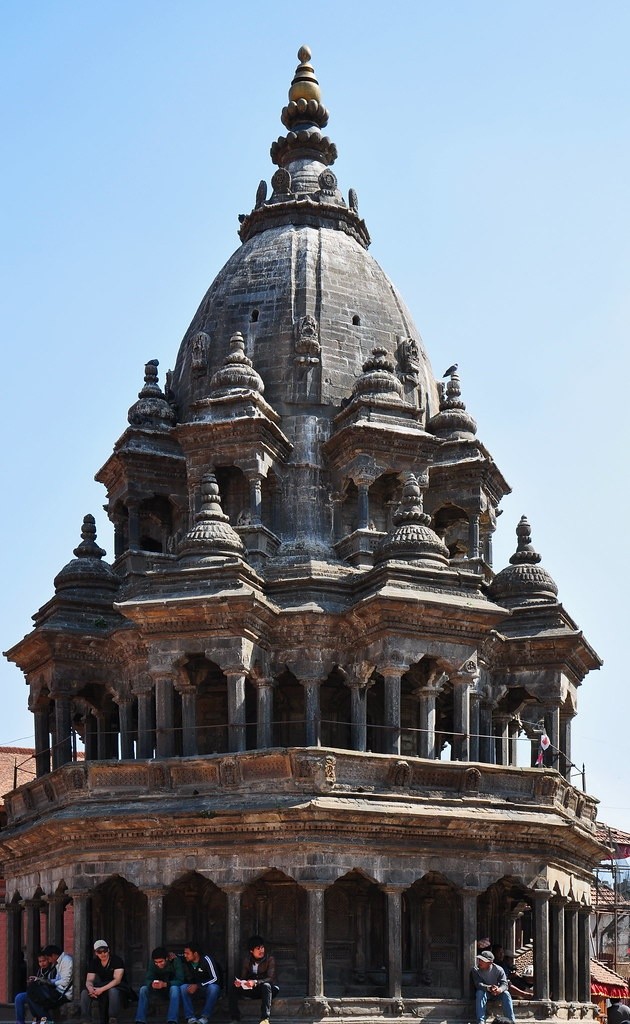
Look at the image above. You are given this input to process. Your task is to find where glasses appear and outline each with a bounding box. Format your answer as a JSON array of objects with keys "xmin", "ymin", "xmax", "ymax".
[{"xmin": 96, "ymin": 950, "xmax": 108, "ymax": 954}]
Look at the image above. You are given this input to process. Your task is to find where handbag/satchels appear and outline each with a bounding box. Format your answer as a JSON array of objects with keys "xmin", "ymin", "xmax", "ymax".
[
  {"xmin": 27, "ymin": 982, "xmax": 63, "ymax": 1009},
  {"xmin": 491, "ymin": 1016, "xmax": 515, "ymax": 1024}
]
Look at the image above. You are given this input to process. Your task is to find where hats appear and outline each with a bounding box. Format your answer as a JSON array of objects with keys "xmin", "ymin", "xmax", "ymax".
[
  {"xmin": 93, "ymin": 939, "xmax": 108, "ymax": 950},
  {"xmin": 476, "ymin": 950, "xmax": 495, "ymax": 962},
  {"xmin": 504, "ymin": 950, "xmax": 518, "ymax": 958}
]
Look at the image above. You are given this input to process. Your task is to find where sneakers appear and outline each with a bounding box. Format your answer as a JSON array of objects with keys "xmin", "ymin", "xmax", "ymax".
[
  {"xmin": 197, "ymin": 1017, "xmax": 208, "ymax": 1024},
  {"xmin": 188, "ymin": 1017, "xmax": 196, "ymax": 1024}
]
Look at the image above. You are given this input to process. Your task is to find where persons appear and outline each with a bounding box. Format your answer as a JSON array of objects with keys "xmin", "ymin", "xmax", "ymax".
[
  {"xmin": 471, "ymin": 951, "xmax": 515, "ymax": 1024},
  {"xmin": 227, "ymin": 939, "xmax": 279, "ymax": 1024},
  {"xmin": 607, "ymin": 998, "xmax": 630, "ymax": 1024},
  {"xmin": 14, "ymin": 939, "xmax": 224, "ymax": 1024}
]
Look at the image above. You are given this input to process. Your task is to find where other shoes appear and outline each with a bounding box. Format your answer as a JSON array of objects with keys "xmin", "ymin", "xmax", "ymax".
[
  {"xmin": 32, "ymin": 1017, "xmax": 54, "ymax": 1024},
  {"xmin": 229, "ymin": 1019, "xmax": 240, "ymax": 1024},
  {"xmin": 109, "ymin": 1018, "xmax": 117, "ymax": 1024},
  {"xmin": 258, "ymin": 1018, "xmax": 270, "ymax": 1024}
]
